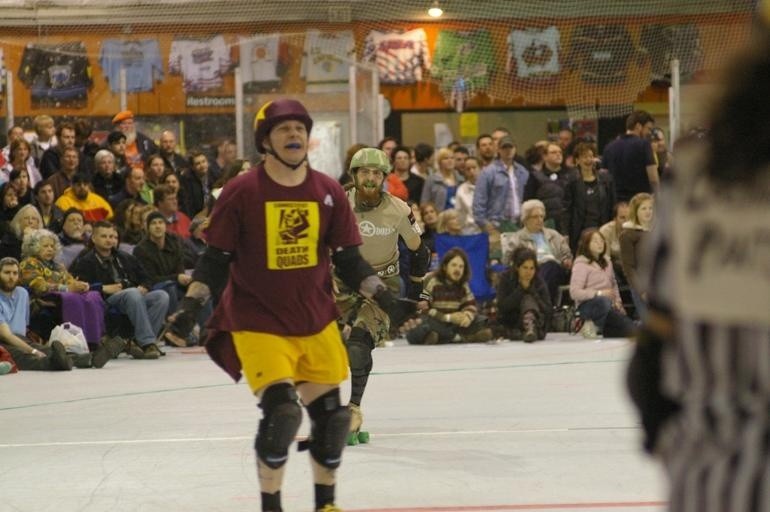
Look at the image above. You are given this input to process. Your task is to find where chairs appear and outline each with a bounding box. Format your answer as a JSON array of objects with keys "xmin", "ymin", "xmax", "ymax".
[
  {"xmin": 502, "ymin": 229, "xmax": 570, "ymax": 305},
  {"xmin": 434, "ymin": 233, "xmax": 496, "ymax": 300}
]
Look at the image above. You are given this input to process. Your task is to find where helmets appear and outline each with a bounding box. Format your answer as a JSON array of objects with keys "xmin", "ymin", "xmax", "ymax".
[
  {"xmin": 252, "ymin": 98, "xmax": 313, "ymax": 154},
  {"xmin": 347, "ymin": 147, "xmax": 392, "ymax": 176}
]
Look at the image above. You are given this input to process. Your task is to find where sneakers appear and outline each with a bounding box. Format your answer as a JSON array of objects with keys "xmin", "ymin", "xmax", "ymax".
[
  {"xmin": 130, "ymin": 344, "xmax": 160, "ymax": 359},
  {"xmin": 91, "ymin": 336, "xmax": 123, "ymax": 368},
  {"xmin": 50, "ymin": 340, "xmax": 73, "ymax": 370},
  {"xmin": 422, "ymin": 323, "xmax": 538, "ymax": 342},
  {"xmin": 584, "ymin": 322, "xmax": 603, "ymax": 338}
]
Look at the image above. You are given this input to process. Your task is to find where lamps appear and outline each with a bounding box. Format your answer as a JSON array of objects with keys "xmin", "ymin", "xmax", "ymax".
[{"xmin": 428, "ymin": 0, "xmax": 443, "ymax": 16}]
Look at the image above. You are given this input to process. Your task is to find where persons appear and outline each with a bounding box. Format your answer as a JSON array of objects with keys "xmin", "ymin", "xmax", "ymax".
[{"xmin": 655, "ymin": 44, "xmax": 770, "ymax": 512}]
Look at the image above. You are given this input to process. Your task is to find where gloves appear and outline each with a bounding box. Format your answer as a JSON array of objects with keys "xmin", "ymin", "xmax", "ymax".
[
  {"xmin": 406, "ymin": 279, "xmax": 424, "ymax": 301},
  {"xmin": 382, "ymin": 300, "xmax": 418, "ymax": 329},
  {"xmin": 165, "ymin": 295, "xmax": 205, "ymax": 341}
]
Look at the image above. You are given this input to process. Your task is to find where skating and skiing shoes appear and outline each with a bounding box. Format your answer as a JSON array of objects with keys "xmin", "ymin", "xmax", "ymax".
[{"xmin": 342, "ymin": 402, "xmax": 371, "ymax": 446}]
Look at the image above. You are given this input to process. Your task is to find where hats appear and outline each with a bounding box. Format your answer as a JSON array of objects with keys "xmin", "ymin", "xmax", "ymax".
[
  {"xmin": 112, "ymin": 111, "xmax": 134, "ymax": 122},
  {"xmin": 146, "ymin": 210, "xmax": 205, "ymax": 229},
  {"xmin": 70, "ymin": 170, "xmax": 91, "ymax": 182},
  {"xmin": 498, "ymin": 136, "xmax": 515, "ymax": 148}
]
